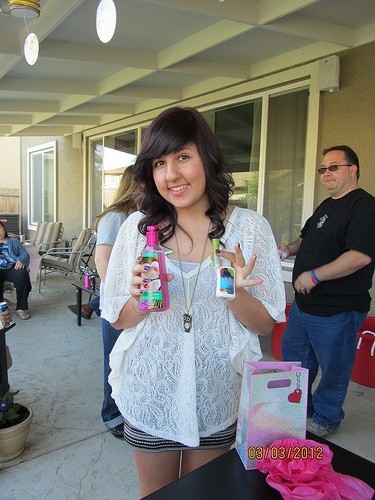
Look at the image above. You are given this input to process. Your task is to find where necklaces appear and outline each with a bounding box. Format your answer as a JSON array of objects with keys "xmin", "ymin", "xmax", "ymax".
[{"xmin": 175, "ymin": 222, "xmax": 212, "ymax": 333}]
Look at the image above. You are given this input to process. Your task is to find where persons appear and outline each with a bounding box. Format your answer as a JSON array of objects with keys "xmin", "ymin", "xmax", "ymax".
[
  {"xmin": 276, "ymin": 146, "xmax": 375, "ymax": 437},
  {"xmin": 93, "ymin": 164, "xmax": 138, "ymax": 440},
  {"xmin": 101, "ymin": 105, "xmax": 288, "ymax": 500},
  {"xmin": 67, "ymin": 296, "xmax": 102, "ymax": 320},
  {"xmin": 0, "ymin": 221, "xmax": 32, "ymax": 368}
]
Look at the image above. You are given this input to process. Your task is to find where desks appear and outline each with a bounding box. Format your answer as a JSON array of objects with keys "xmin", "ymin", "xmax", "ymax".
[
  {"xmin": 138, "ymin": 430, "xmax": 375, "ymax": 500},
  {"xmin": 71, "ymin": 280, "xmax": 99, "ymax": 326}
]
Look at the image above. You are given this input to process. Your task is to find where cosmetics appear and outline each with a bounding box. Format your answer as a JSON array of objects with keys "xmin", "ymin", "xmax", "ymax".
[{"xmin": 216, "ymin": 257, "xmax": 235, "ymax": 301}]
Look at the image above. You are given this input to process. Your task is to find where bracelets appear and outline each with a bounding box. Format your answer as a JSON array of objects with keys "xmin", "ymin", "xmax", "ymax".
[
  {"xmin": 312, "ymin": 270, "xmax": 320, "ymax": 283},
  {"xmin": 310, "ymin": 272, "xmax": 317, "ymax": 285}
]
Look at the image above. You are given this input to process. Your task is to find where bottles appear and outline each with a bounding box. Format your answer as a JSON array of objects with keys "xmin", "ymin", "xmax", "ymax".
[{"xmin": 216, "ymin": 257, "xmax": 236, "ymax": 300}]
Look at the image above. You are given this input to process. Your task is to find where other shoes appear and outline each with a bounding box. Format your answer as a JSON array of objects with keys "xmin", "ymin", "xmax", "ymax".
[
  {"xmin": 68, "ymin": 305, "xmax": 92, "ymax": 319},
  {"xmin": 111, "ymin": 423, "xmax": 124, "ymax": 437},
  {"xmin": 16, "ymin": 310, "xmax": 29, "ymax": 320},
  {"xmin": 306, "ymin": 421, "xmax": 337, "ymax": 437}
]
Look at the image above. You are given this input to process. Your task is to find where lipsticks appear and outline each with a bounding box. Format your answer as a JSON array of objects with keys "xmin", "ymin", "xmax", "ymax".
[{"xmin": 212, "ymin": 239, "xmax": 220, "ymax": 267}]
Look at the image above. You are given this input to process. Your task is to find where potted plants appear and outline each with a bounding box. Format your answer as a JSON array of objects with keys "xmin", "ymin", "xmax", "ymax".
[{"xmin": 0, "ymin": 390, "xmax": 33, "ymax": 461}]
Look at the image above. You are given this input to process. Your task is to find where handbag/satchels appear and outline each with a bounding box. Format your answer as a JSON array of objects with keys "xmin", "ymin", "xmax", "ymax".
[{"xmin": 234, "ymin": 360, "xmax": 309, "ymax": 471}]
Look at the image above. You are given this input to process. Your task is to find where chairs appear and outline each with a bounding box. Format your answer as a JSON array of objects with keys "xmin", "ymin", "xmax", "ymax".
[{"xmin": 3, "ymin": 221, "xmax": 97, "ymax": 292}]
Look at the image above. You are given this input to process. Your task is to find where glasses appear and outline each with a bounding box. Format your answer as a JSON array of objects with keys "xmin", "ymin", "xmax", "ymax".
[{"xmin": 318, "ymin": 164, "xmax": 352, "ymax": 174}]
